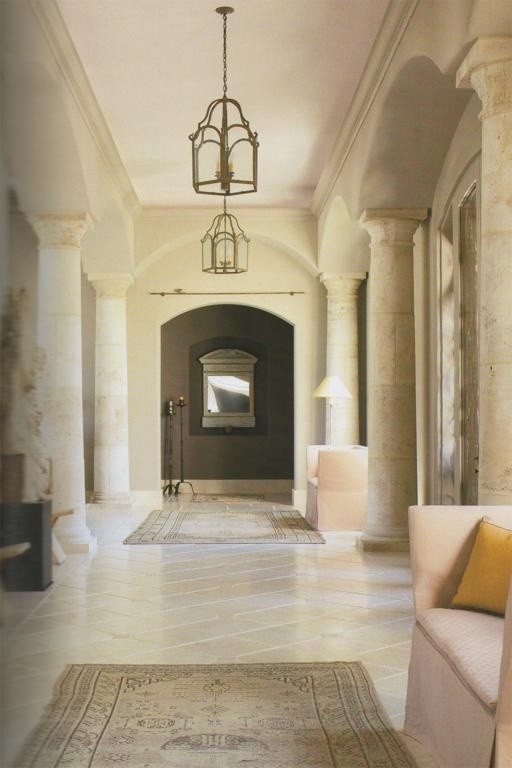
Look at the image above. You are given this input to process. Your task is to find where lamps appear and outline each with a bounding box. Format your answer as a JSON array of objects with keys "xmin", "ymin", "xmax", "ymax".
[
  {"xmin": 189, "ymin": 6, "xmax": 258, "ymax": 277},
  {"xmin": 313, "ymin": 375, "xmax": 352, "ymax": 445}
]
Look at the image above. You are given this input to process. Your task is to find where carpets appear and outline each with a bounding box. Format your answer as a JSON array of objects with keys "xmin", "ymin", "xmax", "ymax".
[
  {"xmin": 15, "ymin": 662, "xmax": 416, "ymax": 767},
  {"xmin": 122, "ymin": 507, "xmax": 327, "ymax": 545},
  {"xmin": 192, "ymin": 492, "xmax": 269, "ymax": 502}
]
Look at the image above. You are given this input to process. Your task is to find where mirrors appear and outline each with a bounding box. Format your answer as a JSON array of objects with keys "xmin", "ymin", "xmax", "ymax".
[{"xmin": 197, "ymin": 348, "xmax": 258, "ymax": 428}]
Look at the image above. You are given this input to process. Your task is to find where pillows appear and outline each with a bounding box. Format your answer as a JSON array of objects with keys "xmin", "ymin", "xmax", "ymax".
[{"xmin": 448, "ymin": 518, "xmax": 511, "ymax": 617}]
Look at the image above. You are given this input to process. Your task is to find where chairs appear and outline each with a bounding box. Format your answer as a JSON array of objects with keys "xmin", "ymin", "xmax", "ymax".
[{"xmin": 305, "ymin": 445, "xmax": 367, "ymax": 532}]
